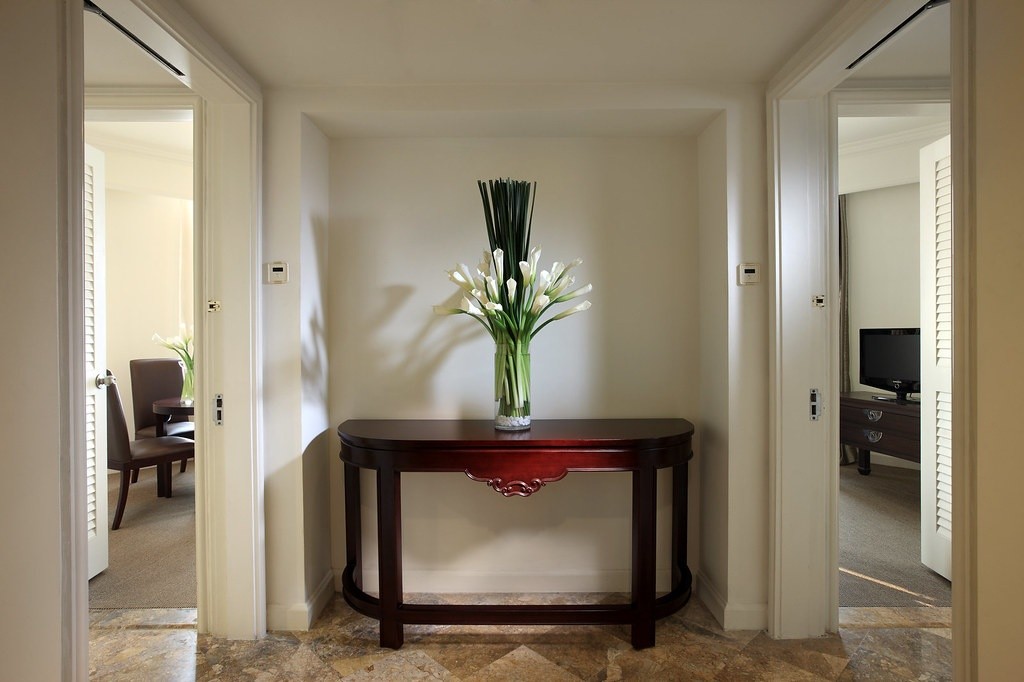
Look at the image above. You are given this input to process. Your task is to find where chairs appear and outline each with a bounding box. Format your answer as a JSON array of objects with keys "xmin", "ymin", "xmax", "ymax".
[
  {"xmin": 130, "ymin": 359, "xmax": 190, "ymax": 484},
  {"xmin": 106, "ymin": 370, "xmax": 194, "ymax": 530}
]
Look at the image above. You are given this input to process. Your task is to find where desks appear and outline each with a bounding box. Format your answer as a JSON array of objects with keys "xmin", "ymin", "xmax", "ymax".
[
  {"xmin": 838, "ymin": 392, "xmax": 921, "ymax": 475},
  {"xmin": 154, "ymin": 398, "xmax": 194, "ymax": 499},
  {"xmin": 336, "ymin": 418, "xmax": 696, "ymax": 650}
]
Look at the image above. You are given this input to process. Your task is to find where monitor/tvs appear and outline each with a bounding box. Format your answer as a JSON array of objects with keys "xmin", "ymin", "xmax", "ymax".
[{"xmin": 859, "ymin": 328, "xmax": 921, "ymax": 406}]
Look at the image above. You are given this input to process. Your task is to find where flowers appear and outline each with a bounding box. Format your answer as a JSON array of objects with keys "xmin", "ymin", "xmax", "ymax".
[
  {"xmin": 433, "ymin": 178, "xmax": 591, "ymax": 418},
  {"xmin": 152, "ymin": 324, "xmax": 196, "ymax": 370}
]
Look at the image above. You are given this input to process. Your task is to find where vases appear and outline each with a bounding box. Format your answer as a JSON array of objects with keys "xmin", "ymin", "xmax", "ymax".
[
  {"xmin": 494, "ymin": 354, "xmax": 531, "ymax": 430},
  {"xmin": 180, "ymin": 371, "xmax": 194, "ymax": 405}
]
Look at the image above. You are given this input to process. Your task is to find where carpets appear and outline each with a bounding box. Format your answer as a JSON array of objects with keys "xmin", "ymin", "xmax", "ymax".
[{"xmin": 88, "ymin": 457, "xmax": 194, "ymax": 609}]
